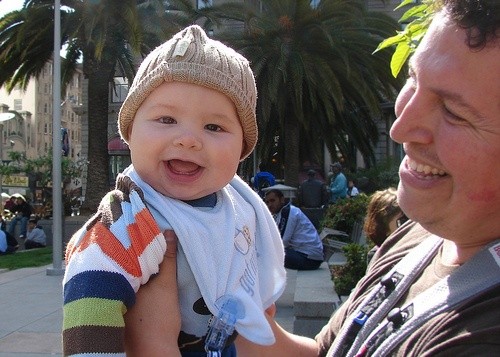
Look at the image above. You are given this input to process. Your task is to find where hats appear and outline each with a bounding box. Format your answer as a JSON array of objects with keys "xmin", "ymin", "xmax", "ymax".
[
  {"xmin": 306, "ymin": 169, "xmax": 316, "ymax": 176},
  {"xmin": 330, "ymin": 162, "xmax": 342, "ymax": 169},
  {"xmin": 117, "ymin": 24, "xmax": 259, "ymax": 162}
]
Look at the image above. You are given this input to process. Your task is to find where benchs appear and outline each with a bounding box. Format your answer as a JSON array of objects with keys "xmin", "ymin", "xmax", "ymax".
[{"xmin": 293, "ymin": 262, "xmax": 342, "ymax": 338}]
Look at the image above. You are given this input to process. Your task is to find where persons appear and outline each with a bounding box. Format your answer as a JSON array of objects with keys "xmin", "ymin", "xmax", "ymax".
[
  {"xmin": 0, "ymin": 196, "xmax": 47, "ymax": 255},
  {"xmin": 62, "ymin": 23, "xmax": 286, "ymax": 357},
  {"xmin": 250, "ymin": 159, "xmax": 410, "ymax": 271},
  {"xmin": 122, "ymin": 0, "xmax": 500, "ymax": 357}
]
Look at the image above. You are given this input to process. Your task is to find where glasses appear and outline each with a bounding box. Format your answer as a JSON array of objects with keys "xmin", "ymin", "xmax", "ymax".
[{"xmin": 391, "ymin": 215, "xmax": 409, "ymax": 227}]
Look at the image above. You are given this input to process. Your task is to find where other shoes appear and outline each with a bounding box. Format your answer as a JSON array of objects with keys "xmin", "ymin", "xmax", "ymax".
[{"xmin": 19, "ymin": 235, "xmax": 23, "ymax": 238}]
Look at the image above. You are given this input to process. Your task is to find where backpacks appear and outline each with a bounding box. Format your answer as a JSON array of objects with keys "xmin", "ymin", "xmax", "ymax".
[{"xmin": 257, "ymin": 176, "xmax": 271, "ymax": 190}]
